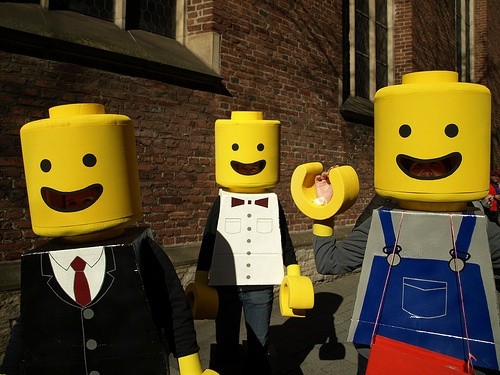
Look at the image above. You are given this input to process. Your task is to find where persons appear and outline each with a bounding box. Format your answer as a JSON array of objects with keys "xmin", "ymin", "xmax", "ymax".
[
  {"xmin": 185, "ymin": 111, "xmax": 314, "ymax": 375},
  {"xmin": 1, "ymin": 103, "xmax": 219, "ymax": 375},
  {"xmin": 290, "ymin": 71, "xmax": 500, "ymax": 375}
]
